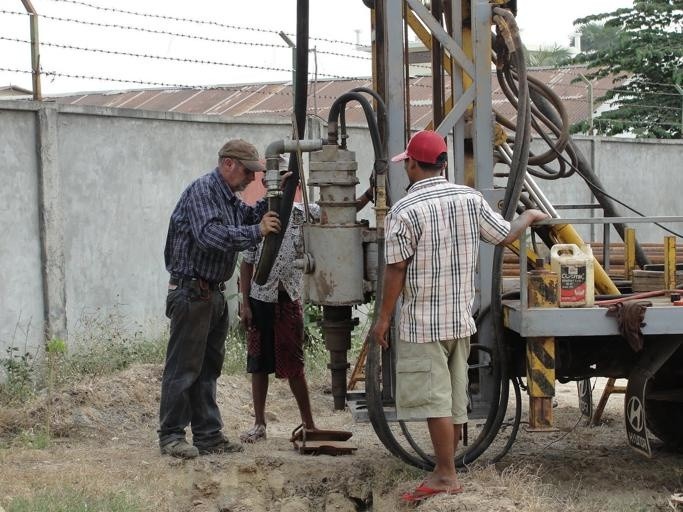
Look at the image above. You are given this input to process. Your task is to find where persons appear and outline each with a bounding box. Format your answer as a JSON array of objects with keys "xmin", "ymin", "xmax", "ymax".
[
  {"xmin": 370, "ymin": 130, "xmax": 550, "ymax": 501},
  {"xmin": 157, "ymin": 139, "xmax": 301, "ymax": 459},
  {"xmin": 239, "ymin": 153, "xmax": 319, "ymax": 443}
]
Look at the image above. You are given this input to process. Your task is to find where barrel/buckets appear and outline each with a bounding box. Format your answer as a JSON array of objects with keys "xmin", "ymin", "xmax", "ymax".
[{"xmin": 550, "ymin": 242, "xmax": 597, "ymax": 308}]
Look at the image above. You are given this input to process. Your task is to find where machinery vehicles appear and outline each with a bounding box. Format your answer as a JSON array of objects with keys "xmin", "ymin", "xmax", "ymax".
[{"xmin": 252, "ymin": 0, "xmax": 683, "ymax": 458}]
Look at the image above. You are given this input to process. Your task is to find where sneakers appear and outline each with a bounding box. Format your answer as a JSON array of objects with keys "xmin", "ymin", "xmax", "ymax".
[{"xmin": 160, "ymin": 433, "xmax": 246, "ymax": 459}]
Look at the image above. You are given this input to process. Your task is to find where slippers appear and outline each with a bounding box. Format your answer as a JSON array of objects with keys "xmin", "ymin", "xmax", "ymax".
[
  {"xmin": 400, "ymin": 477, "xmax": 464, "ymax": 509},
  {"xmin": 239, "ymin": 424, "xmax": 267, "ymax": 445}
]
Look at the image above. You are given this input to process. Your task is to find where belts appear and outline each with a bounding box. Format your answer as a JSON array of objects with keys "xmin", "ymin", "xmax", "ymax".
[{"xmin": 169, "ymin": 277, "xmax": 227, "ymax": 293}]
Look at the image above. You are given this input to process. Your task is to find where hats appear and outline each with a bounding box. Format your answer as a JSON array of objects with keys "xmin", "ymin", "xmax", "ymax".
[
  {"xmin": 218, "ymin": 140, "xmax": 290, "ymax": 172},
  {"xmin": 391, "ymin": 130, "xmax": 448, "ymax": 164}
]
